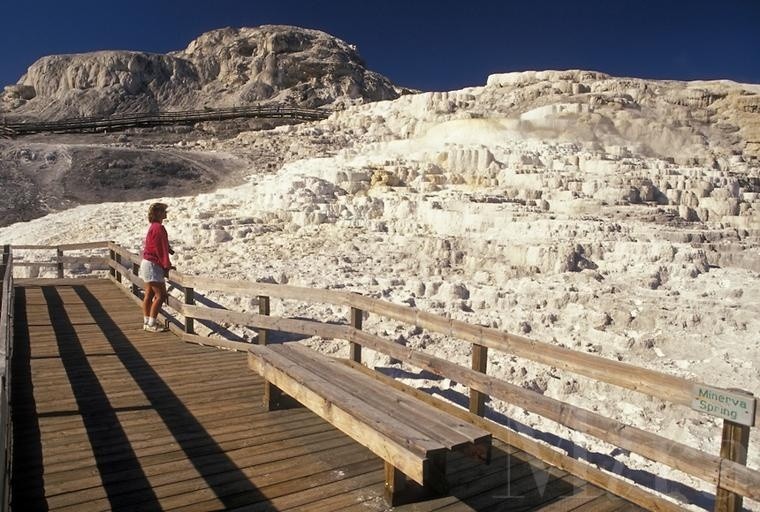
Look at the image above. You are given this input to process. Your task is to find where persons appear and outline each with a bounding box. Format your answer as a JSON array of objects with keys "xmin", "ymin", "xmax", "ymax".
[{"xmin": 138, "ymin": 202, "xmax": 171, "ymax": 333}]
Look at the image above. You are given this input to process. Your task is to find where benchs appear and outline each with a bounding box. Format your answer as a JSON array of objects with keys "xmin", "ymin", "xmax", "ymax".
[{"xmin": 246, "ymin": 341, "xmax": 493, "ymax": 508}]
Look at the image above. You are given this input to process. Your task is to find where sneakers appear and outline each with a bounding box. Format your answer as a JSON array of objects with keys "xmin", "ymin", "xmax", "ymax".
[
  {"xmin": 146, "ymin": 323, "xmax": 164, "ymax": 332},
  {"xmin": 143, "ymin": 324, "xmax": 147, "ymax": 330}
]
